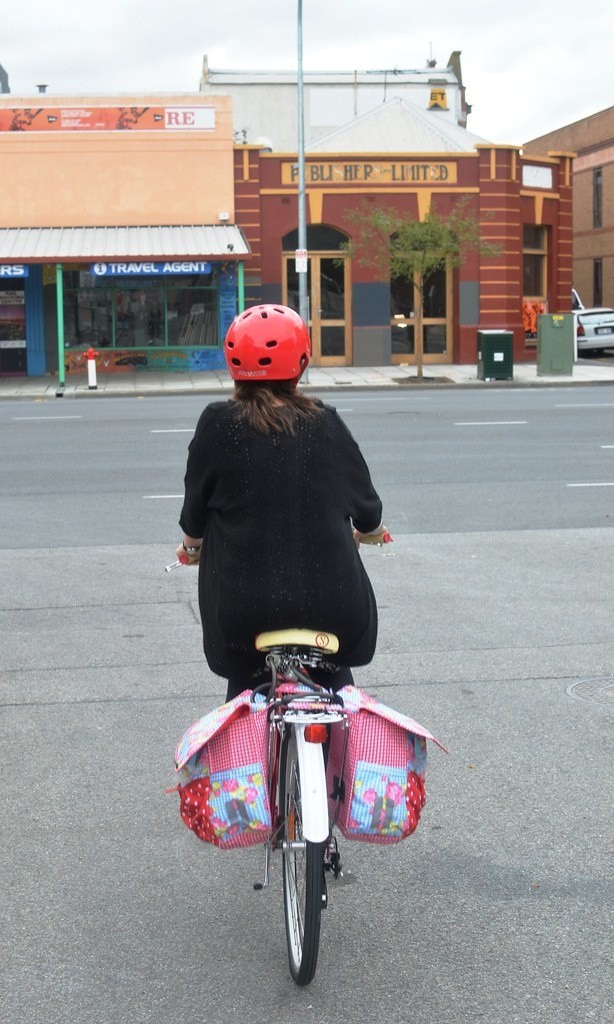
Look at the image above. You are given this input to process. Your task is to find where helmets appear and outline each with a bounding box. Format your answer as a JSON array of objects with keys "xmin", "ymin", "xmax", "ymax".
[{"xmin": 224, "ymin": 303, "xmax": 310, "ymax": 381}]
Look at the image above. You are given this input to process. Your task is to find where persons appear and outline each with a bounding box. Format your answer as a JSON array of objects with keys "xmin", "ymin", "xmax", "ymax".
[{"xmin": 175, "ymin": 303, "xmax": 397, "ymax": 705}]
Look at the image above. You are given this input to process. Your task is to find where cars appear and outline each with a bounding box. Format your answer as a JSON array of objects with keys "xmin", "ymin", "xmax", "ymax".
[{"xmin": 574, "ymin": 307, "xmax": 614, "ymax": 356}]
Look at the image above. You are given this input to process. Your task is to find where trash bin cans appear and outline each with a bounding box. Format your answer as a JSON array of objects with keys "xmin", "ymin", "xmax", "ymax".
[{"xmin": 476, "ymin": 328, "xmax": 513, "ymax": 382}]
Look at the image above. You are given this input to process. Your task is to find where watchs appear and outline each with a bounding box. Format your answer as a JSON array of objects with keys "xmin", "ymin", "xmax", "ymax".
[{"xmin": 183, "ymin": 540, "xmax": 202, "ymax": 556}]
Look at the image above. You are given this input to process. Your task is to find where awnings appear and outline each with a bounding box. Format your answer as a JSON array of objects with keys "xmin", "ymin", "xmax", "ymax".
[{"xmin": 0, "ymin": 225, "xmax": 253, "ymax": 264}]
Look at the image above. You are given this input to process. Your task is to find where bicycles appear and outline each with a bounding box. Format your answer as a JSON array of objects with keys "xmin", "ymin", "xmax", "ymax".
[{"xmin": 164, "ymin": 532, "xmax": 396, "ymax": 986}]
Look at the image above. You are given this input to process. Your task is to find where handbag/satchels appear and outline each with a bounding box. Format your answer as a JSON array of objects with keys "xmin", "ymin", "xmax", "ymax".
[
  {"xmin": 327, "ymin": 684, "xmax": 448, "ymax": 845},
  {"xmin": 171, "ymin": 688, "xmax": 273, "ymax": 851}
]
